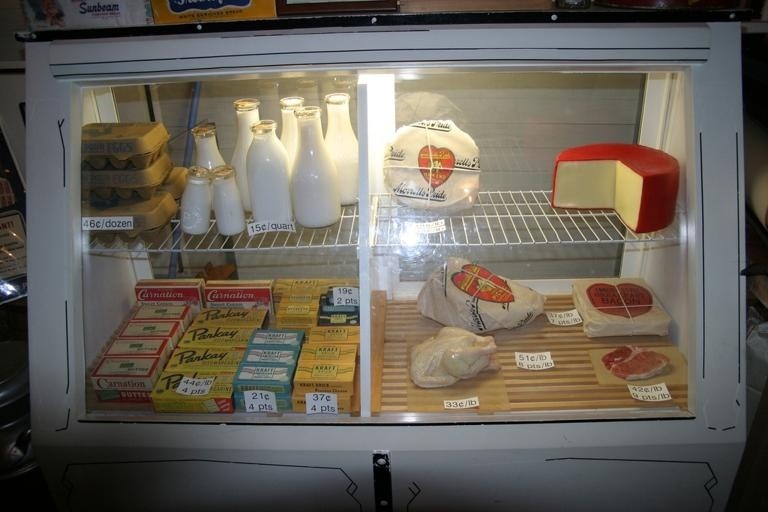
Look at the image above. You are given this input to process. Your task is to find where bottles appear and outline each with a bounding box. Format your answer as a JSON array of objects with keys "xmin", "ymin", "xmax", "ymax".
[
  {"xmin": 191, "ymin": 92, "xmax": 359, "ymax": 212},
  {"xmin": 290, "ymin": 104, "xmax": 341, "ymax": 229},
  {"xmin": 179, "ymin": 166, "xmax": 211, "ymax": 236},
  {"xmin": 211, "ymin": 165, "xmax": 248, "ymax": 238},
  {"xmin": 248, "ymin": 120, "xmax": 291, "ymax": 226}
]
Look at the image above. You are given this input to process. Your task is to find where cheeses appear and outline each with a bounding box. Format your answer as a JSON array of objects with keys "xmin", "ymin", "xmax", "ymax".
[{"xmin": 551, "ymin": 142, "xmax": 679, "ymax": 233}]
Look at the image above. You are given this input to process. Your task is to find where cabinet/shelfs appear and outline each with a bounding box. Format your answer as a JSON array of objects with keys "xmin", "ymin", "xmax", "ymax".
[{"xmin": 23, "ymin": 18, "xmax": 749, "ymax": 512}]
